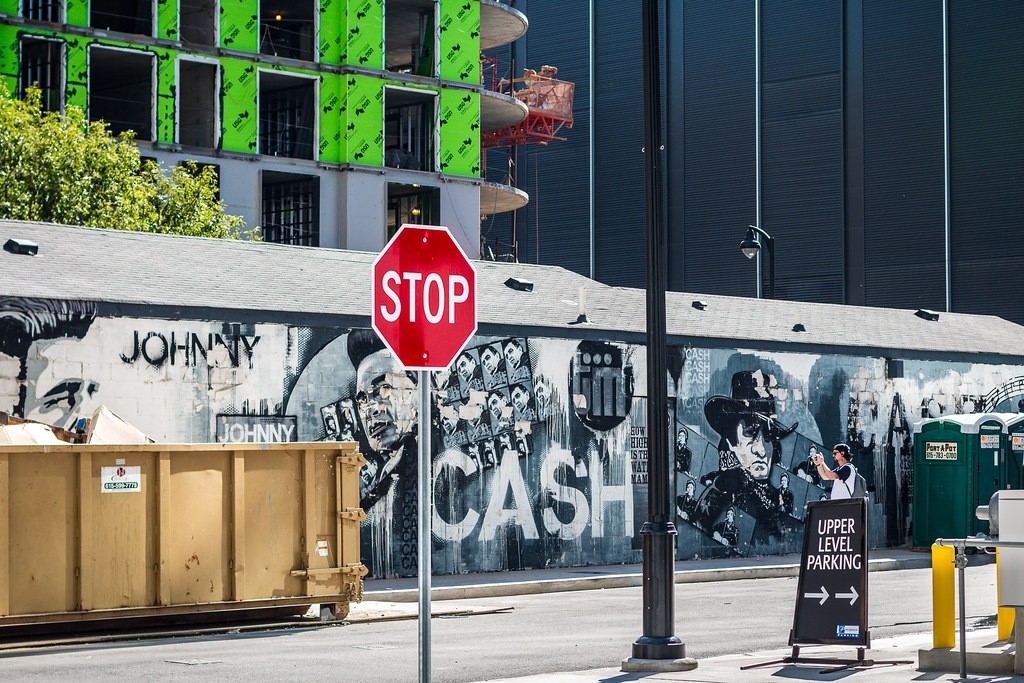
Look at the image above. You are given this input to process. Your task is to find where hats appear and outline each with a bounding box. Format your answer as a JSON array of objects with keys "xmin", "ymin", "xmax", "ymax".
[{"xmin": 834, "ymin": 443, "xmax": 853, "ymax": 456}]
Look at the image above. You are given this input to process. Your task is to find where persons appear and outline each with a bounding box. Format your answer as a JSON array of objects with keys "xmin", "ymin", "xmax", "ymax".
[{"xmin": 813, "ymin": 444, "xmax": 856, "ymax": 499}]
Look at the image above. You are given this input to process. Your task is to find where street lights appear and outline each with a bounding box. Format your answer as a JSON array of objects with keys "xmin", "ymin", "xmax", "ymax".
[{"xmin": 739, "ymin": 225, "xmax": 776, "ymax": 299}]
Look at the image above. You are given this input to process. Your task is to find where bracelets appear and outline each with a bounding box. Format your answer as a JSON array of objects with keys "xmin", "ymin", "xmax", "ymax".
[{"xmin": 816, "ymin": 464, "xmax": 820, "ymax": 466}]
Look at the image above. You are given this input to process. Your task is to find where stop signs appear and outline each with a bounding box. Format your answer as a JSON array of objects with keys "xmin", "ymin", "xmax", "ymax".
[{"xmin": 371, "ymin": 224, "xmax": 478, "ymax": 370}]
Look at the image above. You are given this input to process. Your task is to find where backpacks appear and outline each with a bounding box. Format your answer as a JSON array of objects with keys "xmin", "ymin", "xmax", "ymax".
[{"xmin": 839, "ymin": 463, "xmax": 867, "ymax": 498}]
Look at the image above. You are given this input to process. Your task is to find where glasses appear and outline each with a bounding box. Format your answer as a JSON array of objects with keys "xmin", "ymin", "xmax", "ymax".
[{"xmin": 832, "ymin": 451, "xmax": 839, "ymax": 454}]
